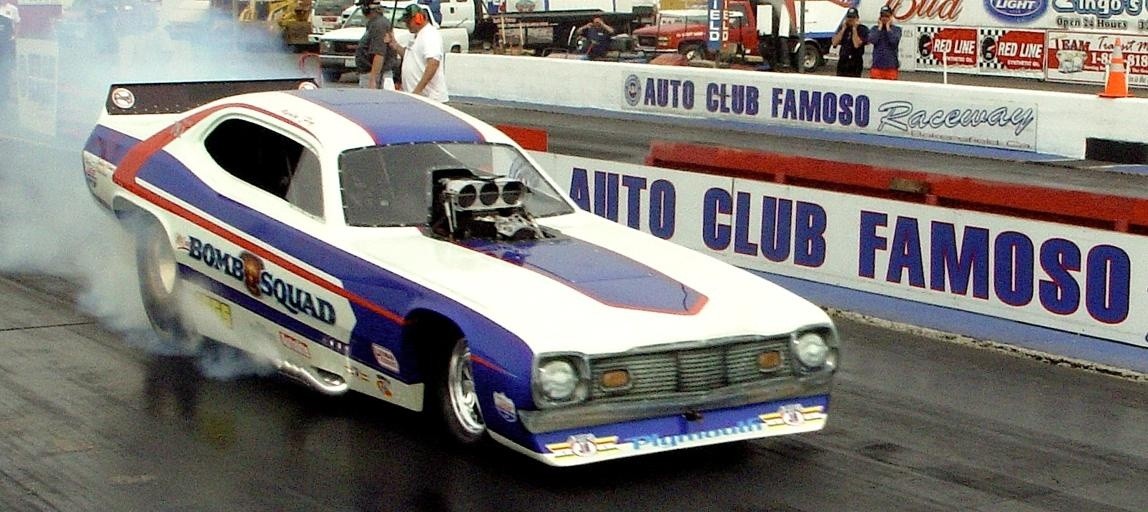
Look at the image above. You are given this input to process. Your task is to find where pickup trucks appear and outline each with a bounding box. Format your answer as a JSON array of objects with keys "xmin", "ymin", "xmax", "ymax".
[{"xmin": 317, "ymin": 1, "xmax": 472, "ymax": 83}]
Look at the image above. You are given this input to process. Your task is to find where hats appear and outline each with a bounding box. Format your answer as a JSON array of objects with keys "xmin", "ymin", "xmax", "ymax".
[
  {"xmin": 847, "ymin": 8, "xmax": 858, "ymax": 18},
  {"xmin": 397, "ymin": 4, "xmax": 424, "ymax": 23},
  {"xmin": 880, "ymin": 6, "xmax": 892, "ymax": 14}
]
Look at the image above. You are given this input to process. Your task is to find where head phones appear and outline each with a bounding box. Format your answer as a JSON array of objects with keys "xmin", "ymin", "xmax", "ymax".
[
  {"xmin": 415, "ymin": 13, "xmax": 425, "ymax": 24},
  {"xmin": 362, "ymin": 0, "xmax": 370, "ymax": 16}
]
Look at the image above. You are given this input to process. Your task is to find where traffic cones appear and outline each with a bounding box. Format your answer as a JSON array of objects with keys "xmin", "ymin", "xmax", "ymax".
[{"xmin": 1096, "ymin": 37, "xmax": 1137, "ymax": 99}]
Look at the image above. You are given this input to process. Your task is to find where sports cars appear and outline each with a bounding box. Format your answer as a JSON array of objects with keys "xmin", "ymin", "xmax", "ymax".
[{"xmin": 75, "ymin": 81, "xmax": 843, "ymax": 470}]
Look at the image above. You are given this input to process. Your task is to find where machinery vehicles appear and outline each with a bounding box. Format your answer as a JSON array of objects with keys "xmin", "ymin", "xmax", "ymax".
[{"xmin": 229, "ymin": 0, "xmax": 316, "ymax": 52}]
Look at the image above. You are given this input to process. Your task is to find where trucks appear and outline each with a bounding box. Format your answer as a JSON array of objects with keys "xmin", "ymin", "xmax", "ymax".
[{"xmin": 633, "ymin": 0, "xmax": 855, "ymax": 72}]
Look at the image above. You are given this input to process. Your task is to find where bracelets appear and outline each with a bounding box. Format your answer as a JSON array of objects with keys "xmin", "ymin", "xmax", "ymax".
[{"xmin": 391, "ymin": 41, "xmax": 400, "ymax": 51}]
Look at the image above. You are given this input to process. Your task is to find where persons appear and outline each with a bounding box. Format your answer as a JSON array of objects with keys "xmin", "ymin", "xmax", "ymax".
[
  {"xmin": 576, "ymin": 17, "xmax": 617, "ymax": 61},
  {"xmin": 865, "ymin": 4, "xmax": 903, "ymax": 81},
  {"xmin": 829, "ymin": 6, "xmax": 870, "ymax": 78},
  {"xmin": 351, "ymin": 0, "xmax": 398, "ymax": 90},
  {"xmin": 0, "ymin": 0, "xmax": 22, "ymax": 105},
  {"xmin": 278, "ymin": 4, "xmax": 314, "ymax": 45},
  {"xmin": 382, "ymin": 2, "xmax": 451, "ymax": 106}
]
